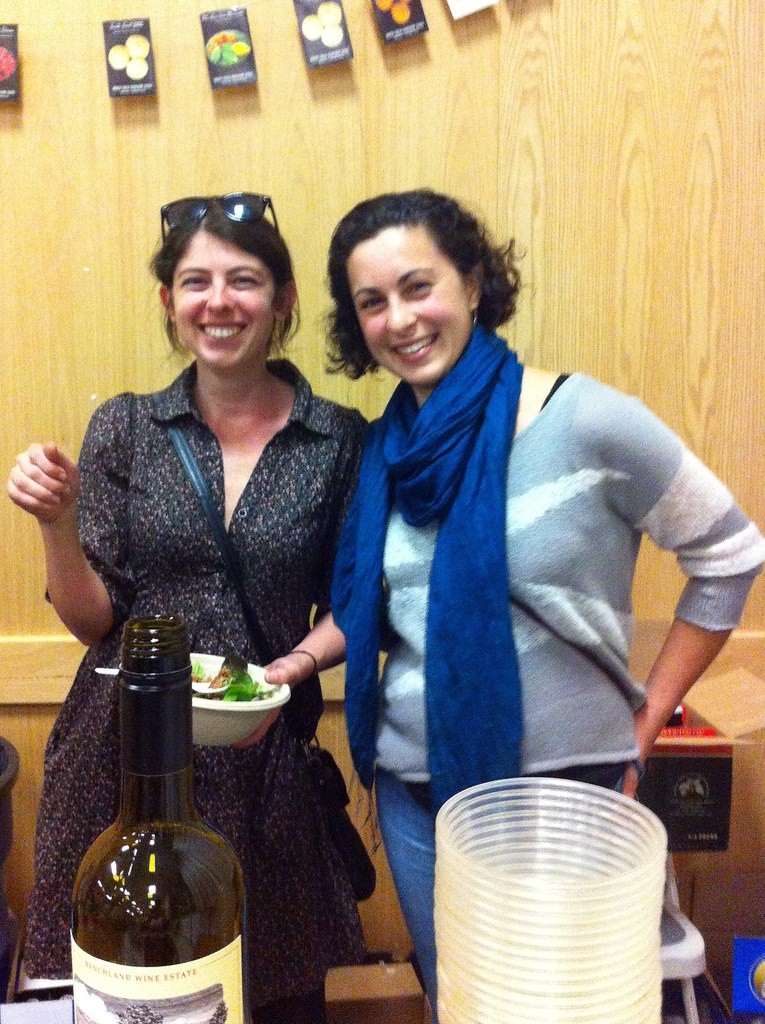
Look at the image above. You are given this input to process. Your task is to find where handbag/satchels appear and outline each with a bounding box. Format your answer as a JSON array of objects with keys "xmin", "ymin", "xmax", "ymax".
[{"xmin": 305, "ymin": 745, "xmax": 376, "ymax": 902}]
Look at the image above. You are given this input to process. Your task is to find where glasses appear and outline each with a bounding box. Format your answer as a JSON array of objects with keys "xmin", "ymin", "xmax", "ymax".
[{"xmin": 160, "ymin": 193, "xmax": 279, "ymax": 245}]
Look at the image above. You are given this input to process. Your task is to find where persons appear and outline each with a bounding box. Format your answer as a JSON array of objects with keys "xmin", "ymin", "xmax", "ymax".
[
  {"xmin": 325, "ymin": 186, "xmax": 765, "ymax": 1024},
  {"xmin": 6, "ymin": 199, "xmax": 369, "ymax": 1024}
]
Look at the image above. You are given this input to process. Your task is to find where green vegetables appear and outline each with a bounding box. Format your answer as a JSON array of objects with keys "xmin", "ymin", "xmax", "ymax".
[{"xmin": 190, "ymin": 664, "xmax": 273, "ymax": 701}]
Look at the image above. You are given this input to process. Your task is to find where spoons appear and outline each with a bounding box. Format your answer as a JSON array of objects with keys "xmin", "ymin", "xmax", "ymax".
[{"xmin": 94, "ymin": 666, "xmax": 232, "ymax": 695}]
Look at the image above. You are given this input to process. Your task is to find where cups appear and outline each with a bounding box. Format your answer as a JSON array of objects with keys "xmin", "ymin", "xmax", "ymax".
[{"xmin": 434, "ymin": 777, "xmax": 669, "ymax": 1024}]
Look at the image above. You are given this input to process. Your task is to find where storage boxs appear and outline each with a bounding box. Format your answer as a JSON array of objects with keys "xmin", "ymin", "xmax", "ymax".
[
  {"xmin": 325, "ymin": 962, "xmax": 426, "ymax": 1024},
  {"xmin": 639, "ymin": 698, "xmax": 733, "ymax": 851}
]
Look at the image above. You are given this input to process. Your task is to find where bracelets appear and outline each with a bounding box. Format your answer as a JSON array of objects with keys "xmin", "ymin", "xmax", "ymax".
[
  {"xmin": 290, "ymin": 651, "xmax": 316, "ymax": 677},
  {"xmin": 633, "ymin": 758, "xmax": 645, "ymax": 778}
]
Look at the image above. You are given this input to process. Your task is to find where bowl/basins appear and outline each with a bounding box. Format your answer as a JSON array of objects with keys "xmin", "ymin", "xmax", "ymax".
[{"xmin": 190, "ymin": 652, "xmax": 291, "ymax": 746}]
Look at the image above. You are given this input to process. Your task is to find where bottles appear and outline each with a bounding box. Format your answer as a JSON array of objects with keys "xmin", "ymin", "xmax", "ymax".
[{"xmin": 69, "ymin": 616, "xmax": 250, "ymax": 1024}]
